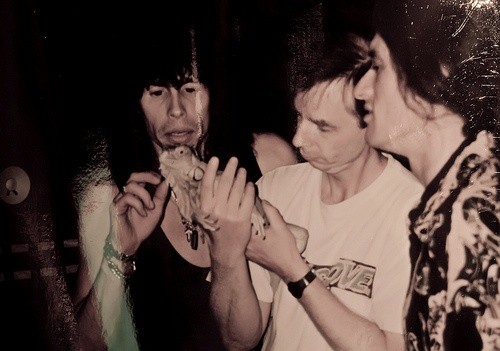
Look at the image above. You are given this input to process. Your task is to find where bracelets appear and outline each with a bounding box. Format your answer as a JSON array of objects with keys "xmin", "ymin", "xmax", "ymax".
[
  {"xmin": 105, "ymin": 240, "xmax": 138, "ymax": 263},
  {"xmin": 105, "ymin": 255, "xmax": 133, "ymax": 279}
]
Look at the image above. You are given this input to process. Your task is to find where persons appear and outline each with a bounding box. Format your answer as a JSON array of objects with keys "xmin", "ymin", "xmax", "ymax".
[
  {"xmin": 73, "ymin": 26, "xmax": 275, "ymax": 351},
  {"xmin": 355, "ymin": 10, "xmax": 499, "ymax": 351},
  {"xmin": 194, "ymin": 44, "xmax": 426, "ymax": 351}
]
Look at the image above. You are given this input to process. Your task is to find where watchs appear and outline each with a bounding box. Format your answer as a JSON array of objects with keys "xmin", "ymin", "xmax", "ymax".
[{"xmin": 287, "ymin": 271, "xmax": 317, "ymax": 299}]
